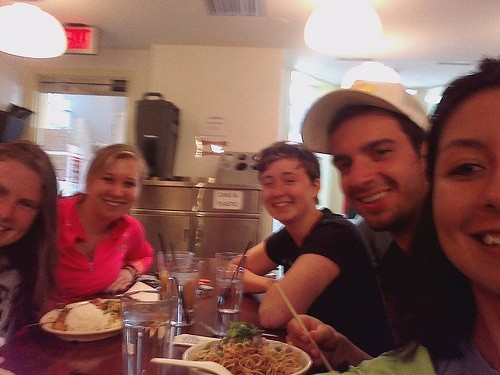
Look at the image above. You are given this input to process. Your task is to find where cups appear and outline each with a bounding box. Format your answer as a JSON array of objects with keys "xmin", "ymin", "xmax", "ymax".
[
  {"xmin": 120, "ymin": 289, "xmax": 179, "ymax": 375},
  {"xmin": 174, "ymin": 270, "xmax": 200, "ymax": 327},
  {"xmin": 214, "ymin": 251, "xmax": 247, "ymax": 314},
  {"xmin": 157, "ymin": 250, "xmax": 195, "ymax": 301}
]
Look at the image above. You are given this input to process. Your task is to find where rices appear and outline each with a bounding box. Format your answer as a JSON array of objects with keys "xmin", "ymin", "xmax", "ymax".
[{"xmin": 64, "ymin": 304, "xmax": 110, "ymax": 332}]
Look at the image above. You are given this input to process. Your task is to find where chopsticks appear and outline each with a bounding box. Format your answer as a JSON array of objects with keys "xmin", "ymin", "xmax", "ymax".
[
  {"xmin": 157, "ymin": 232, "xmax": 190, "ymax": 325},
  {"xmin": 275, "ymin": 282, "xmax": 333, "ymax": 372},
  {"xmin": 218, "ymin": 241, "xmax": 253, "ymax": 306}
]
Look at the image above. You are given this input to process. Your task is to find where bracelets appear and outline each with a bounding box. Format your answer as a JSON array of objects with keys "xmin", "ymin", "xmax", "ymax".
[{"xmin": 123, "ymin": 266, "xmax": 136, "ymax": 278}]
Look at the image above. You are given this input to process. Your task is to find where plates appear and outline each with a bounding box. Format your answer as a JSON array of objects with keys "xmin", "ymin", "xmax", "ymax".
[
  {"xmin": 39, "ymin": 298, "xmax": 123, "ymax": 342},
  {"xmin": 181, "ymin": 338, "xmax": 313, "ymax": 375}
]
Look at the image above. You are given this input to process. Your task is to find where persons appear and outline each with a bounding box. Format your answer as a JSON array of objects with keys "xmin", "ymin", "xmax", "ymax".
[
  {"xmin": 300, "ymin": 79, "xmax": 477, "ymax": 365},
  {"xmin": 284, "ymin": 58, "xmax": 500, "ymax": 375},
  {"xmin": 227, "ymin": 141, "xmax": 397, "ymax": 358},
  {"xmin": 30, "ymin": 143, "xmax": 156, "ymax": 328},
  {"xmin": 0, "ymin": 140, "xmax": 58, "ymax": 347}
]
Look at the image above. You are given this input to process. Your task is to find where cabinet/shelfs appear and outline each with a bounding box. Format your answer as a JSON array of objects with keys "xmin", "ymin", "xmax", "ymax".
[{"xmin": 129, "ymin": 181, "xmax": 274, "ymax": 258}]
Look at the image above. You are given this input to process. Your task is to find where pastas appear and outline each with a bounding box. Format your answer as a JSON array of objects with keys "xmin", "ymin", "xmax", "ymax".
[{"xmin": 196, "ymin": 336, "xmax": 302, "ymax": 375}]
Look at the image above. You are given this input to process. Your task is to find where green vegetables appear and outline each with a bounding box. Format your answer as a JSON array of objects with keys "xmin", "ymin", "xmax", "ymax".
[{"xmin": 220, "ymin": 322, "xmax": 265, "ymax": 345}]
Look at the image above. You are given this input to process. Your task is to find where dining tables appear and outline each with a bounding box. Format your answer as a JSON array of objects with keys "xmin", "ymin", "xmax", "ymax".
[{"xmin": 0, "ymin": 259, "xmax": 285, "ymax": 375}]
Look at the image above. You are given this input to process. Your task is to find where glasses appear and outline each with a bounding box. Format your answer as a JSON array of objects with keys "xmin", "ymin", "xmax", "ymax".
[{"xmin": 253, "ymin": 142, "xmax": 301, "ymax": 161}]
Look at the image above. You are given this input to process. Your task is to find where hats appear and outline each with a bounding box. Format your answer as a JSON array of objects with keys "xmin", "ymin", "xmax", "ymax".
[{"xmin": 301, "ymin": 80, "xmax": 431, "ymax": 153}]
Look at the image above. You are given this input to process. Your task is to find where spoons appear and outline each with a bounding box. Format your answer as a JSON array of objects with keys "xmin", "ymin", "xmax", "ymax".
[{"xmin": 151, "ymin": 358, "xmax": 233, "ymax": 375}]
